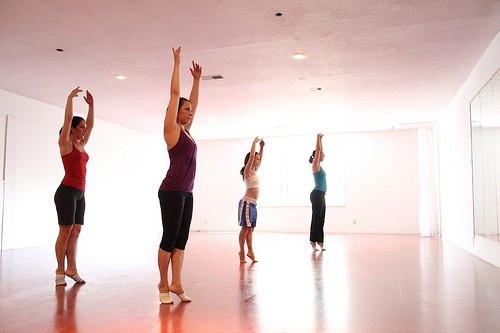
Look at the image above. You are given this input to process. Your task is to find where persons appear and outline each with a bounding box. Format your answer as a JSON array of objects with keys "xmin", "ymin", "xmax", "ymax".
[
  {"xmin": 237, "ymin": 135, "xmax": 265, "ymax": 262},
  {"xmin": 308, "ymin": 133, "xmax": 327, "ymax": 252},
  {"xmin": 157, "ymin": 45, "xmax": 202, "ymax": 303},
  {"xmin": 54, "ymin": 84, "xmax": 93, "ymax": 287}
]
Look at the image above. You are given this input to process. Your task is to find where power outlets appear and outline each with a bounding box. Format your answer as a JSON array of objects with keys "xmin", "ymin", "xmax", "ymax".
[{"xmin": 352, "ymin": 219, "xmax": 356, "ymax": 225}]
